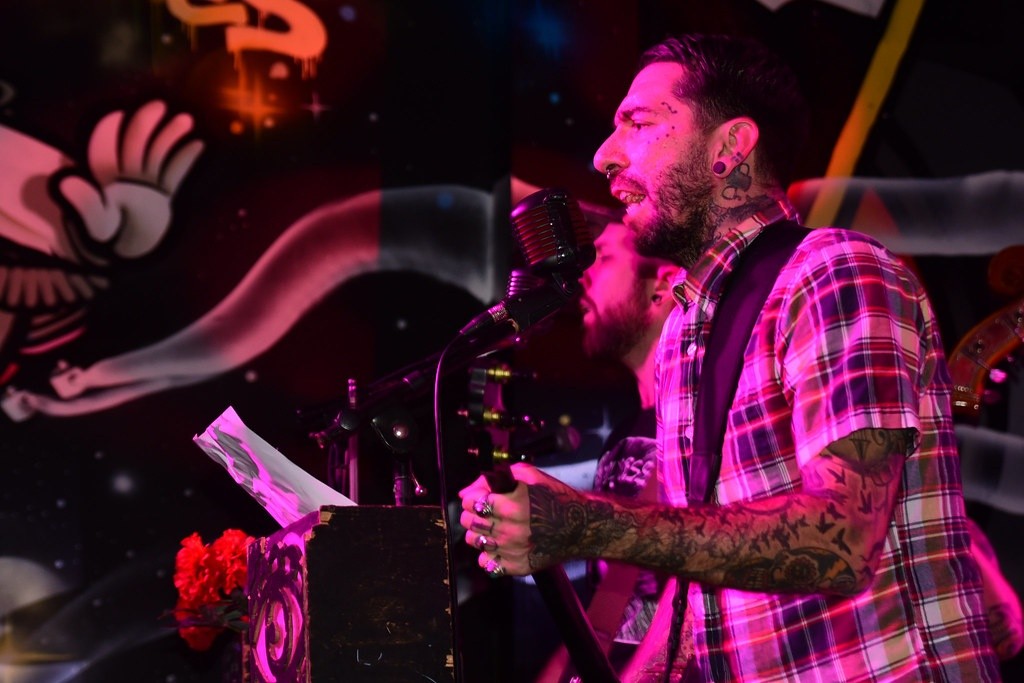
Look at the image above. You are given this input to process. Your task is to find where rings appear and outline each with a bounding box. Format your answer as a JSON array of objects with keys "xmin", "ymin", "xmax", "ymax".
[
  {"xmin": 471, "ymin": 533, "xmax": 500, "ymax": 553},
  {"xmin": 473, "ymin": 490, "xmax": 497, "ymax": 516},
  {"xmin": 468, "ymin": 512, "xmax": 496, "ymax": 536},
  {"xmin": 479, "ymin": 555, "xmax": 508, "ymax": 577}
]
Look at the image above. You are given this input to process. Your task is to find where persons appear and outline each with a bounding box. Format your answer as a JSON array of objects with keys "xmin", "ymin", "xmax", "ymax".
[
  {"xmin": 531, "ymin": 205, "xmax": 696, "ymax": 683},
  {"xmin": 457, "ymin": 36, "xmax": 1022, "ymax": 682}
]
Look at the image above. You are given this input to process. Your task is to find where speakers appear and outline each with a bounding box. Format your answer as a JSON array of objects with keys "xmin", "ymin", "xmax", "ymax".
[{"xmin": 247, "ymin": 505, "xmax": 455, "ymax": 683}]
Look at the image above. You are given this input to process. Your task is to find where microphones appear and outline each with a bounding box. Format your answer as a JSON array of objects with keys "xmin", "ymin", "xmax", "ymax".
[
  {"xmin": 505, "ymin": 269, "xmax": 545, "ymax": 298},
  {"xmin": 458, "ymin": 185, "xmax": 597, "ymax": 337}
]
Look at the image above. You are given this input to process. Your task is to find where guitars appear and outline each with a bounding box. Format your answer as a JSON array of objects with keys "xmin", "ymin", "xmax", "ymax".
[{"xmin": 452, "ymin": 346, "xmax": 637, "ymax": 683}]
